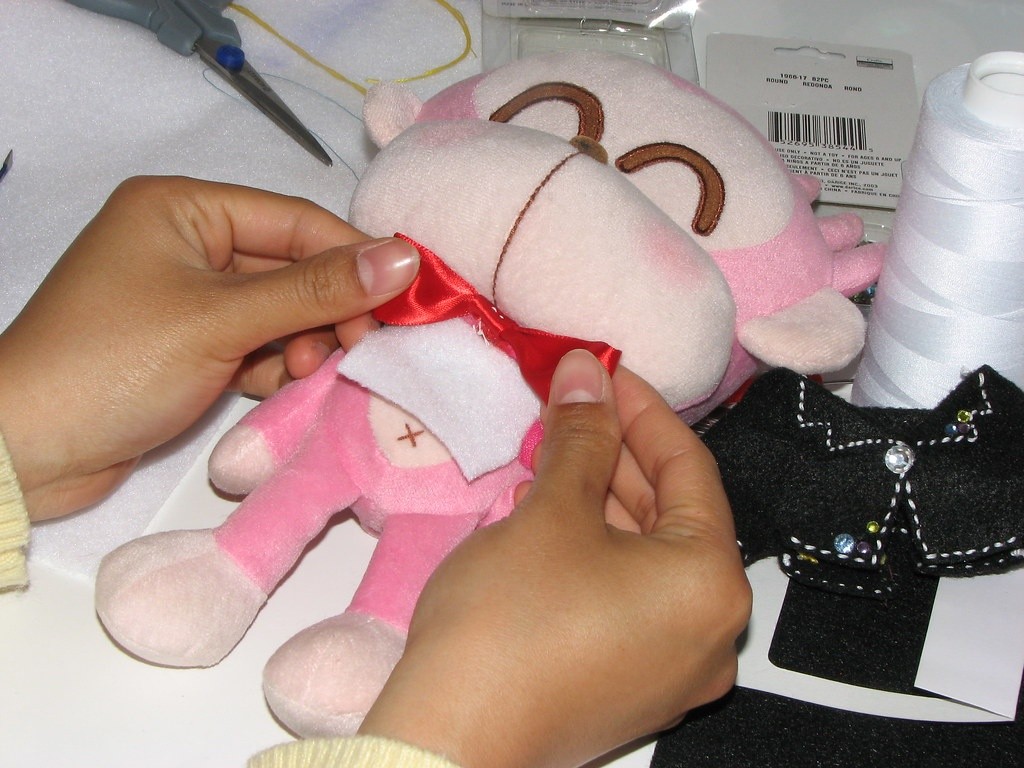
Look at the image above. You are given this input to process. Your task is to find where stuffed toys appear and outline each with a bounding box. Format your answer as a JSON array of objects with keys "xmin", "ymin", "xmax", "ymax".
[{"xmin": 93, "ymin": 42, "xmax": 891, "ymax": 744}]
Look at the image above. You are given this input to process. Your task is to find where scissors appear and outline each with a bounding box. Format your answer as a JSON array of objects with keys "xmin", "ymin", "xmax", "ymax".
[{"xmin": 64, "ymin": 1, "xmax": 336, "ymax": 168}]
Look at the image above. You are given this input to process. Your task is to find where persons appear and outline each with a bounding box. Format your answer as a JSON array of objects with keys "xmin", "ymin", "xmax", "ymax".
[{"xmin": 2, "ymin": 171, "xmax": 755, "ymax": 768}]
